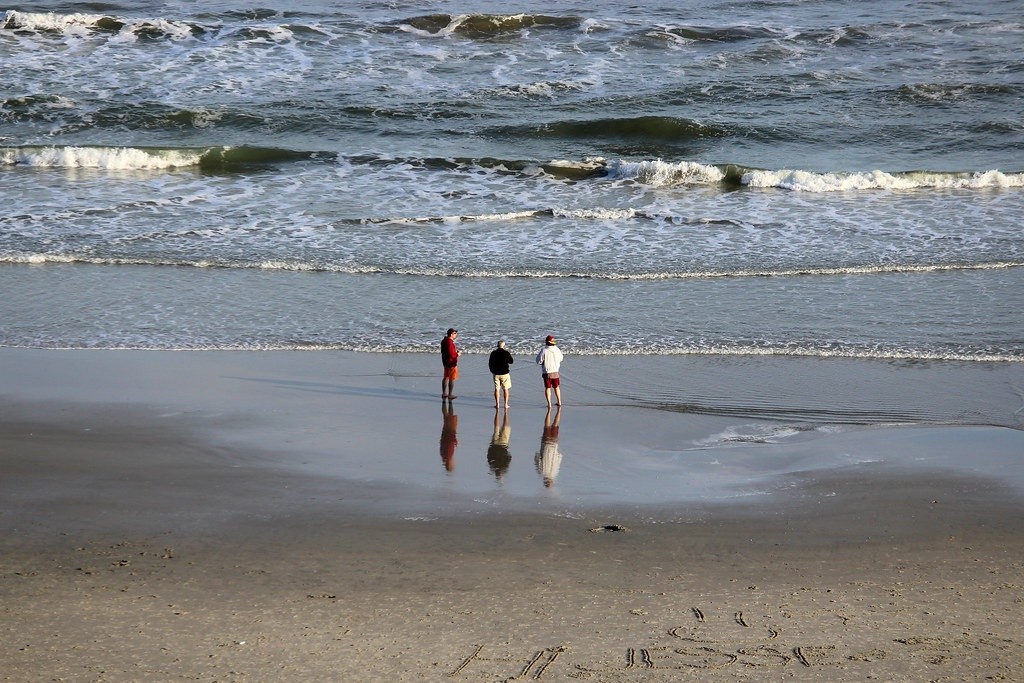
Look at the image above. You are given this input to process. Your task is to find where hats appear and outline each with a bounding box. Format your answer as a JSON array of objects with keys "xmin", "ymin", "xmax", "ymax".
[
  {"xmin": 447, "ymin": 328, "xmax": 458, "ymax": 335},
  {"xmin": 546, "ymin": 335, "xmax": 556, "ymax": 345}
]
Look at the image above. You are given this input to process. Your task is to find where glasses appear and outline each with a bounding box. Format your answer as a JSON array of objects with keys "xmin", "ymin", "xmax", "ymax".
[{"xmin": 453, "ymin": 332, "xmax": 456, "ymax": 334}]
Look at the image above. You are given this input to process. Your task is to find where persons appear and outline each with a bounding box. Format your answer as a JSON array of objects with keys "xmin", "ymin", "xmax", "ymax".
[
  {"xmin": 489, "ymin": 341, "xmax": 513, "ymax": 408},
  {"xmin": 536, "ymin": 335, "xmax": 563, "ymax": 407},
  {"xmin": 534, "ymin": 407, "xmax": 563, "ymax": 487},
  {"xmin": 441, "ymin": 328, "xmax": 462, "ymax": 398},
  {"xmin": 440, "ymin": 399, "xmax": 457, "ymax": 472},
  {"xmin": 487, "ymin": 408, "xmax": 512, "ymax": 478}
]
